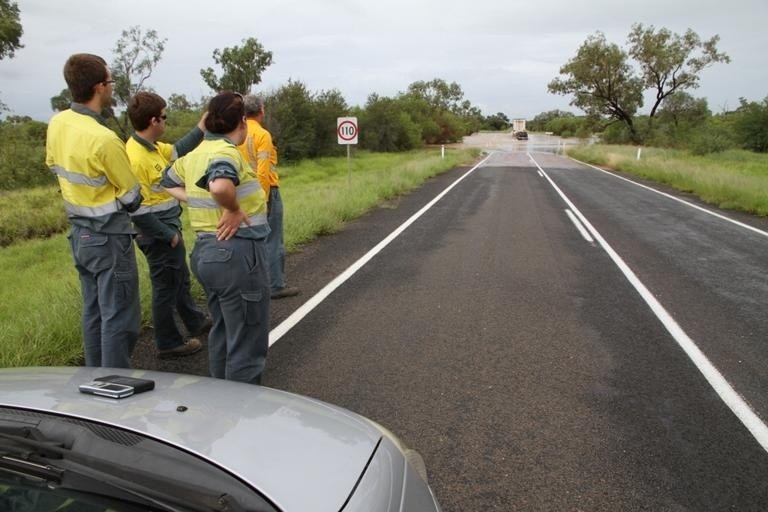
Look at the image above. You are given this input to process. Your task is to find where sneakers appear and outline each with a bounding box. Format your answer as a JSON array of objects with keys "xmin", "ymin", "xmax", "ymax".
[
  {"xmin": 156, "ymin": 338, "xmax": 202, "ymax": 359},
  {"xmin": 270, "ymin": 286, "xmax": 300, "ymax": 299},
  {"xmin": 190, "ymin": 314, "xmax": 212, "ymax": 339}
]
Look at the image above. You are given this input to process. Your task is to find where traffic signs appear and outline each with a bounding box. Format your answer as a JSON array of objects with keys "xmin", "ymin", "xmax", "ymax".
[{"xmin": 335, "ymin": 115, "xmax": 358, "ymax": 146}]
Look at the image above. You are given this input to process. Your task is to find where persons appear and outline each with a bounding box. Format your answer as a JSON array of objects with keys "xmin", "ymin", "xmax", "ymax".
[
  {"xmin": 234, "ymin": 94, "xmax": 299, "ymax": 299},
  {"xmin": 158, "ymin": 92, "xmax": 271, "ymax": 385},
  {"xmin": 44, "ymin": 54, "xmax": 142, "ymax": 370},
  {"xmin": 124, "ymin": 92, "xmax": 209, "ymax": 358}
]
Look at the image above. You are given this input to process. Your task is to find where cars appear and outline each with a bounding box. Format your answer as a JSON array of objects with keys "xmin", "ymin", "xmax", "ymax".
[
  {"xmin": 515, "ymin": 131, "xmax": 528, "ymax": 140},
  {"xmin": 0, "ymin": 359, "xmax": 445, "ymax": 512}
]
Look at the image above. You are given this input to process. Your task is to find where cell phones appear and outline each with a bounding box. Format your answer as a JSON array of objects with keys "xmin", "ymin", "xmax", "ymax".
[
  {"xmin": 94, "ymin": 375, "xmax": 154, "ymax": 394},
  {"xmin": 79, "ymin": 380, "xmax": 135, "ymax": 399}
]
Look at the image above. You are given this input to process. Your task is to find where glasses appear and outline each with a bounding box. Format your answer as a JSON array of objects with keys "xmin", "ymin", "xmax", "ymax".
[
  {"xmin": 160, "ymin": 114, "xmax": 168, "ymax": 121},
  {"xmin": 101, "ymin": 79, "xmax": 116, "ymax": 88}
]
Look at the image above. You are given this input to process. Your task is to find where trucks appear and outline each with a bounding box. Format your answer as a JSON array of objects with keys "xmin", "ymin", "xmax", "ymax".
[{"xmin": 511, "ymin": 119, "xmax": 526, "ymax": 137}]
